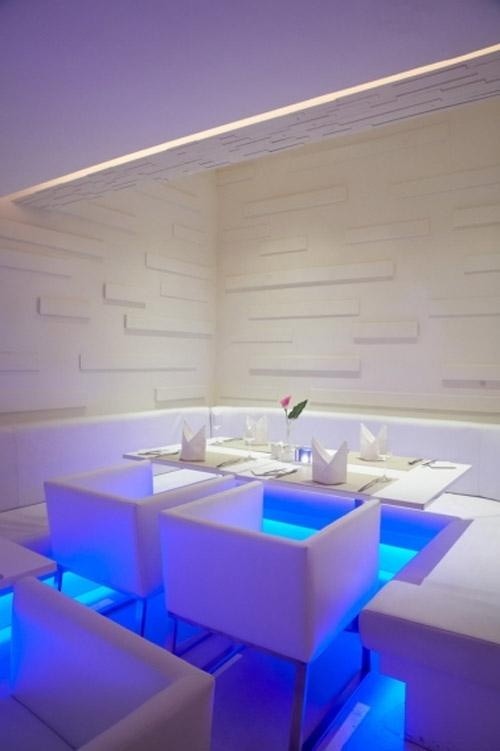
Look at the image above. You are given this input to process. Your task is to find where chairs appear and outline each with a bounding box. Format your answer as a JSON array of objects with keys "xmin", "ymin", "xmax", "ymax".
[
  {"xmin": 159, "ymin": 481, "xmax": 380, "ymax": 751},
  {"xmin": 43, "ymin": 459, "xmax": 236, "ymax": 658},
  {"xmin": 0, "ymin": 576, "xmax": 215, "ymax": 751}
]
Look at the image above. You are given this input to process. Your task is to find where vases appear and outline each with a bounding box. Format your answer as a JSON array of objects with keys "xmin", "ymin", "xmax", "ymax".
[{"xmin": 282, "ymin": 423, "xmax": 296, "ymax": 462}]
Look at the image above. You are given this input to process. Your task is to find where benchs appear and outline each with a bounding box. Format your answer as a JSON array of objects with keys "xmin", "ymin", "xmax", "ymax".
[
  {"xmin": 211, "ymin": 406, "xmax": 500, "ymax": 551},
  {"xmin": 0, "ymin": 406, "xmax": 211, "ymax": 598},
  {"xmin": 359, "ymin": 515, "xmax": 500, "ymax": 751}
]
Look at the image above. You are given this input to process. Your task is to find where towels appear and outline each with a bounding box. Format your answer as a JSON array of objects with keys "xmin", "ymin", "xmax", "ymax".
[
  {"xmin": 244, "ymin": 415, "xmax": 267, "ymax": 445},
  {"xmin": 356, "ymin": 422, "xmax": 388, "ymax": 461},
  {"xmin": 310, "ymin": 436, "xmax": 349, "ymax": 485},
  {"xmin": 179, "ymin": 417, "xmax": 207, "ymax": 461}
]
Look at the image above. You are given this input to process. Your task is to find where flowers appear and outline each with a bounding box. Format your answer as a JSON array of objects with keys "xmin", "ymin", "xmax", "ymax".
[{"xmin": 280, "ymin": 396, "xmax": 309, "ymax": 453}]
